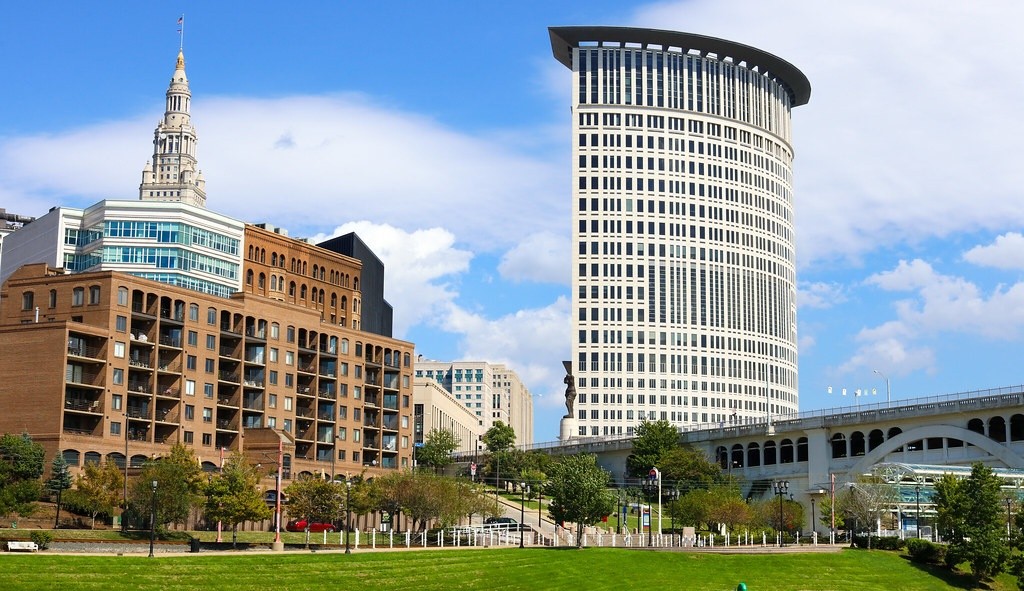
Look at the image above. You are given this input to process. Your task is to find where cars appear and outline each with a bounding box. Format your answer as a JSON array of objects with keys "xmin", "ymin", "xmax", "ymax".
[
  {"xmin": 286, "ymin": 514, "xmax": 337, "ymax": 533},
  {"xmin": 478, "ymin": 517, "xmax": 531, "ymax": 534}
]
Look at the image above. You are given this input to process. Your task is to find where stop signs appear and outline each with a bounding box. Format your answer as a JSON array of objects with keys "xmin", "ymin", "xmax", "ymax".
[
  {"xmin": 650, "ymin": 470, "xmax": 656, "ymax": 476},
  {"xmin": 472, "ymin": 464, "xmax": 476, "ymax": 471}
]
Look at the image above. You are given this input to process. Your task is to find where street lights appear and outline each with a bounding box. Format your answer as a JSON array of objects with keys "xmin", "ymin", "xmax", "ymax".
[
  {"xmin": 637, "ymin": 489, "xmax": 641, "ymax": 533},
  {"xmin": 519, "ymin": 479, "xmax": 527, "ymax": 548},
  {"xmin": 148, "ymin": 477, "xmax": 158, "ymax": 557},
  {"xmin": 55, "ymin": 465, "xmax": 66, "ymax": 528},
  {"xmin": 665, "ymin": 487, "xmax": 680, "ymax": 546},
  {"xmin": 345, "ymin": 479, "xmax": 352, "ymax": 554},
  {"xmin": 1004, "ymin": 494, "xmax": 1012, "ymax": 551},
  {"xmin": 617, "ymin": 485, "xmax": 622, "ymax": 534},
  {"xmin": 538, "ymin": 479, "xmax": 542, "ymax": 528},
  {"xmin": 773, "ymin": 478, "xmax": 790, "ymax": 547},
  {"xmin": 810, "ymin": 496, "xmax": 816, "ymax": 530},
  {"xmin": 208, "ymin": 473, "xmax": 212, "ymax": 501},
  {"xmin": 874, "ymin": 369, "xmax": 891, "ymax": 407},
  {"xmin": 482, "ymin": 480, "xmax": 487, "ymax": 524},
  {"xmin": 641, "ymin": 476, "xmax": 657, "ymax": 549},
  {"xmin": 914, "ymin": 483, "xmax": 921, "ymax": 540}
]
